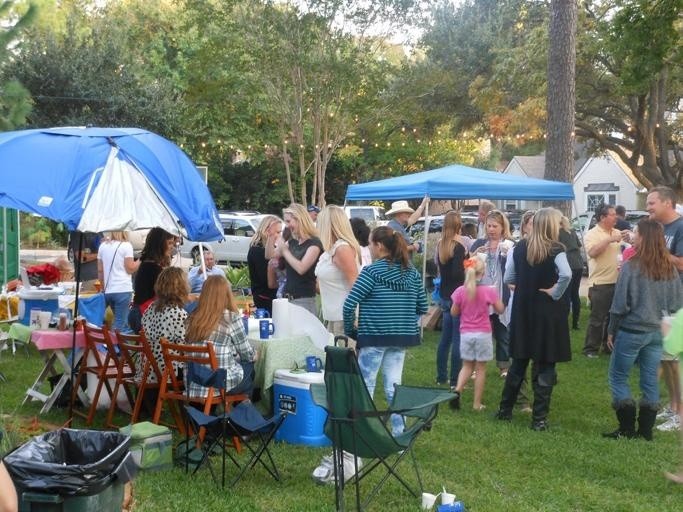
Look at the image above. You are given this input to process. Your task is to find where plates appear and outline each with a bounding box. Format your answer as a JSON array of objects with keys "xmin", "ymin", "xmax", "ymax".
[{"xmin": 36, "ymin": 287, "xmax": 52, "ymax": 290}]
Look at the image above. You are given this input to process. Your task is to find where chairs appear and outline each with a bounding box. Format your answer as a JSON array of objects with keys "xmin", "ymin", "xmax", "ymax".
[
  {"xmin": 66, "ymin": 319, "xmax": 134, "ymax": 417},
  {"xmin": 309, "ymin": 337, "xmax": 459, "ymax": 512},
  {"xmin": 180, "ymin": 365, "xmax": 287, "ymax": 496},
  {"xmin": 151, "ymin": 338, "xmax": 247, "ymax": 458},
  {"xmin": 107, "ymin": 329, "xmax": 181, "ymax": 431}
]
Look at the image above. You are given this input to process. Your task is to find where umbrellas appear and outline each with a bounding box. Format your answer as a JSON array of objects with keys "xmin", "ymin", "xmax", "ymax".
[{"xmin": 0, "ymin": 127, "xmax": 225, "ymax": 428}]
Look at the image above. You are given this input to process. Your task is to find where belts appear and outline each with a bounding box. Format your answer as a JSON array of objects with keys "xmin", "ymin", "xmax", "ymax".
[{"xmin": 282, "ymin": 293, "xmax": 317, "ymax": 301}]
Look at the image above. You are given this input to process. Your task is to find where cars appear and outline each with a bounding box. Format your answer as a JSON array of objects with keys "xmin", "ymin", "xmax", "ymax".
[
  {"xmin": 410, "ymin": 210, "xmax": 537, "ymax": 236},
  {"xmin": 67, "ymin": 228, "xmax": 154, "ymax": 263}
]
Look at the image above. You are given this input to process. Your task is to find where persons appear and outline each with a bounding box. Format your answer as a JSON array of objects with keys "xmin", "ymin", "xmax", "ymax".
[
  {"xmin": 435, "ymin": 200, "xmax": 586, "ymax": 430},
  {"xmin": 248, "ymin": 203, "xmax": 372, "ymax": 351},
  {"xmin": 67, "ymin": 226, "xmax": 224, "ymax": 334},
  {"xmin": 132, "ymin": 266, "xmax": 218, "ymax": 432},
  {"xmin": 584, "ymin": 203, "xmax": 633, "ymax": 357},
  {"xmin": 343, "ymin": 226, "xmax": 428, "ymax": 454},
  {"xmin": 602, "ymin": 219, "xmax": 682, "ymax": 442},
  {"xmin": 385, "ymin": 197, "xmax": 431, "ymax": 257},
  {"xmin": 619, "ymin": 185, "xmax": 682, "ymax": 432},
  {"xmin": 184, "ymin": 276, "xmax": 255, "ymax": 442}
]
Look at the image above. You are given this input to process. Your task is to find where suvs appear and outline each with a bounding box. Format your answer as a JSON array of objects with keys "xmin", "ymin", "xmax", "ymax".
[{"xmin": 179, "ymin": 210, "xmax": 289, "ymax": 267}]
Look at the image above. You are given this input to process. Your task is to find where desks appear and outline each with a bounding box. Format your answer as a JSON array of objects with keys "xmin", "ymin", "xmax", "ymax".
[{"xmin": 5, "ymin": 321, "xmax": 102, "ymax": 418}]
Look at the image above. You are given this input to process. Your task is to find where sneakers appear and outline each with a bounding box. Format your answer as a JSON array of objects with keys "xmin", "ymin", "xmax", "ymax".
[{"xmin": 435, "ymin": 371, "xmax": 683, "ymax": 439}]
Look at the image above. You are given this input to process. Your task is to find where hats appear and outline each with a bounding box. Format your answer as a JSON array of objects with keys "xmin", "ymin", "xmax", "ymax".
[{"xmin": 385, "ymin": 201, "xmax": 416, "ymax": 215}]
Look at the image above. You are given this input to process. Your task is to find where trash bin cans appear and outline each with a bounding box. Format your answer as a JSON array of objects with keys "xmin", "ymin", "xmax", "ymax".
[{"xmin": 3, "ymin": 427, "xmax": 138, "ymax": 512}]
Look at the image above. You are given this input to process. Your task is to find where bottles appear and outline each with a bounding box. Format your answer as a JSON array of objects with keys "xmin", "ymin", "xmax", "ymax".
[{"xmin": 29, "ymin": 308, "xmax": 41, "ymax": 329}]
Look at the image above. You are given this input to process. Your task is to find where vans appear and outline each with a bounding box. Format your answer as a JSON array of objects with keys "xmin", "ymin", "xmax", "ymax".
[
  {"xmin": 337, "ymin": 205, "xmax": 391, "ymax": 228},
  {"xmin": 569, "ymin": 209, "xmax": 650, "ymax": 274}
]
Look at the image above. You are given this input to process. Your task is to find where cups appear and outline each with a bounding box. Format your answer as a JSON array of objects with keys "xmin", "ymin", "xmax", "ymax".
[
  {"xmin": 39, "ymin": 312, "xmax": 51, "ymax": 329},
  {"xmin": 240, "ymin": 317, "xmax": 248, "ymax": 334},
  {"xmin": 94, "ymin": 283, "xmax": 99, "ymax": 293},
  {"xmin": 476, "ymin": 252, "xmax": 486, "ymax": 260},
  {"xmin": 259, "ymin": 320, "xmax": 274, "ymax": 339},
  {"xmin": 76, "ymin": 316, "xmax": 84, "ymax": 331},
  {"xmin": 254, "ymin": 308, "xmax": 270, "ymax": 319},
  {"xmin": 305, "ymin": 355, "xmax": 322, "ymax": 372},
  {"xmin": 662, "ymin": 316, "xmax": 673, "ymax": 326},
  {"xmin": 58, "ymin": 313, "xmax": 66, "ymax": 330},
  {"xmin": 500, "ymin": 239, "xmax": 514, "ymax": 257},
  {"xmin": 420, "ymin": 492, "xmax": 436, "ymax": 510},
  {"xmin": 440, "ymin": 492, "xmax": 455, "ymax": 506},
  {"xmin": 416, "ymin": 241, "xmax": 422, "ymax": 253}
]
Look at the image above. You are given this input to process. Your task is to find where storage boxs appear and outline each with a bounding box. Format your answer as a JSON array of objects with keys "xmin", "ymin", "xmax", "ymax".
[
  {"xmin": 18, "ymin": 282, "xmax": 60, "ymax": 329},
  {"xmin": 119, "ymin": 421, "xmax": 174, "ymax": 469},
  {"xmin": 272, "ymin": 368, "xmax": 335, "ymax": 448}
]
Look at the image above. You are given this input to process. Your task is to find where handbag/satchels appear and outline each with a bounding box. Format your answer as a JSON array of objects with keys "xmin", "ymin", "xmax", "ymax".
[{"xmin": 312, "ymin": 450, "xmax": 362, "ymax": 485}]
